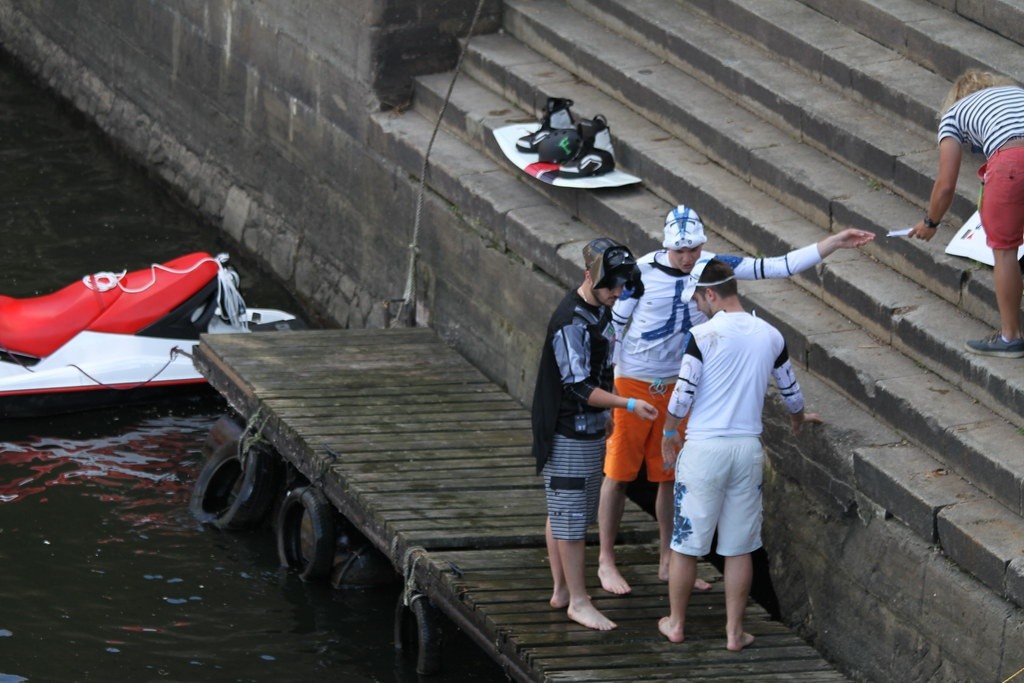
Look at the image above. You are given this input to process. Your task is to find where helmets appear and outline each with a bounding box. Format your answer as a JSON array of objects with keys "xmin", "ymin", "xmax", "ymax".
[{"xmin": 538, "ymin": 128, "xmax": 580, "ymax": 164}]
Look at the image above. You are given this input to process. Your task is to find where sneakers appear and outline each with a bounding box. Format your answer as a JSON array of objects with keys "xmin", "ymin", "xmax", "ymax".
[
  {"xmin": 558, "ymin": 115, "xmax": 615, "ymax": 177},
  {"xmin": 965, "ymin": 328, "xmax": 1024, "ymax": 358},
  {"xmin": 515, "ymin": 97, "xmax": 575, "ymax": 152}
]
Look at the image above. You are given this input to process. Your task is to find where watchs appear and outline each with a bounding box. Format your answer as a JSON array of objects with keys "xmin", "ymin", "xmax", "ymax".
[{"xmin": 924, "ymin": 216, "xmax": 939, "ymax": 229}]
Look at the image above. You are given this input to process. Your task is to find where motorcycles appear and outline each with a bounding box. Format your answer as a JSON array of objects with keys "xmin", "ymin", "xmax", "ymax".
[{"xmin": 0, "ymin": 250, "xmax": 294, "ymax": 421}]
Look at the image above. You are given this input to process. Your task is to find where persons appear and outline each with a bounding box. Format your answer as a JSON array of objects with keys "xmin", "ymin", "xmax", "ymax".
[
  {"xmin": 658, "ymin": 258, "xmax": 824, "ymax": 651},
  {"xmin": 530, "ymin": 238, "xmax": 659, "ymax": 631},
  {"xmin": 597, "ymin": 205, "xmax": 875, "ymax": 593},
  {"xmin": 908, "ymin": 68, "xmax": 1024, "ymax": 358}
]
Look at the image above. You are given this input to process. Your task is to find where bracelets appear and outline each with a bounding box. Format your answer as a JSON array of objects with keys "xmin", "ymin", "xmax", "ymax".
[
  {"xmin": 627, "ymin": 398, "xmax": 635, "ymax": 412},
  {"xmin": 663, "ymin": 429, "xmax": 677, "ymax": 437}
]
